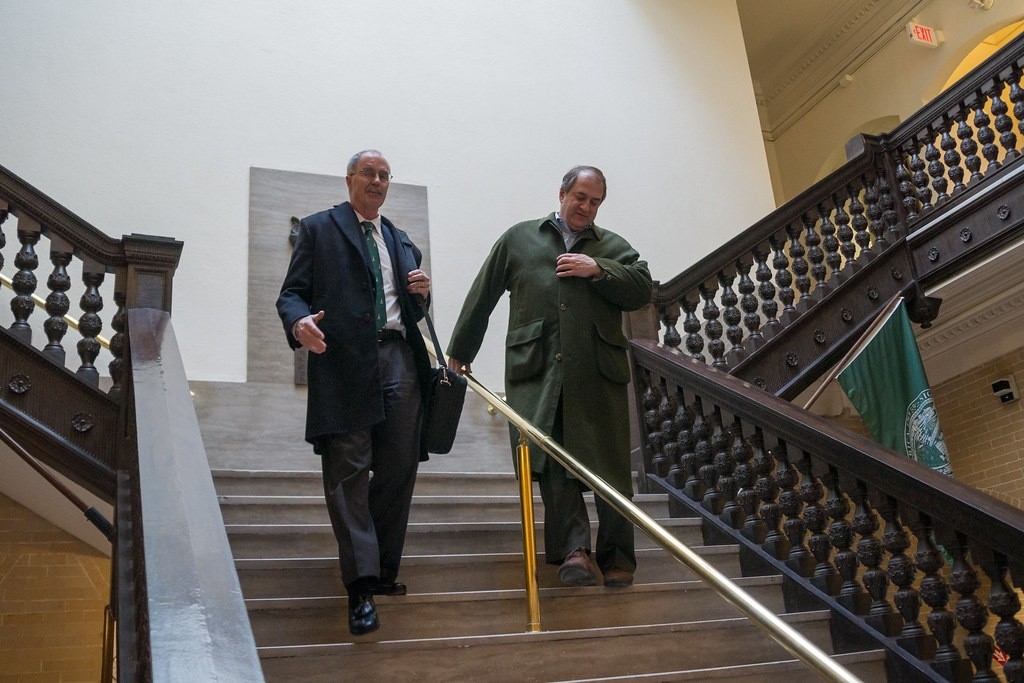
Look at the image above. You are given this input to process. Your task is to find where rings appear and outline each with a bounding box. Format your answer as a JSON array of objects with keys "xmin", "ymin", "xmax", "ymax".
[{"xmin": 420, "ymin": 281, "xmax": 423, "ymax": 287}]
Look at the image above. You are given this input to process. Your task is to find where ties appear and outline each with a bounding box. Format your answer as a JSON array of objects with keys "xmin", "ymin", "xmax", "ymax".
[{"xmin": 361, "ymin": 220, "xmax": 387, "ymax": 332}]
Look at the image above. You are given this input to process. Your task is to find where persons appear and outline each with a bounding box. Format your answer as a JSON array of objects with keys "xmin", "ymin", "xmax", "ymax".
[
  {"xmin": 445, "ymin": 165, "xmax": 654, "ymax": 587},
  {"xmin": 277, "ymin": 150, "xmax": 432, "ymax": 643}
]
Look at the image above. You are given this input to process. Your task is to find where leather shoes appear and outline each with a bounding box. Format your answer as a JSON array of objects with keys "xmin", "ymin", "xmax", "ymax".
[
  {"xmin": 376, "ymin": 580, "xmax": 407, "ymax": 596},
  {"xmin": 348, "ymin": 583, "xmax": 381, "ymax": 635}
]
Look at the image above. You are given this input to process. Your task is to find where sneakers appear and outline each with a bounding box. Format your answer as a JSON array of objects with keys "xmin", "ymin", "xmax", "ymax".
[
  {"xmin": 603, "ymin": 569, "xmax": 634, "ymax": 586},
  {"xmin": 557, "ymin": 546, "xmax": 597, "ymax": 587}
]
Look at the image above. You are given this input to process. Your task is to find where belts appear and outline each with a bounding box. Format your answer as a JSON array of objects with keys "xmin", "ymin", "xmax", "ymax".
[{"xmin": 377, "ymin": 329, "xmax": 404, "ymax": 341}]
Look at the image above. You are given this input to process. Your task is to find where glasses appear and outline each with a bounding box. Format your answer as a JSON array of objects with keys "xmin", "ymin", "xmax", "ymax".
[{"xmin": 350, "ymin": 168, "xmax": 393, "ymax": 181}]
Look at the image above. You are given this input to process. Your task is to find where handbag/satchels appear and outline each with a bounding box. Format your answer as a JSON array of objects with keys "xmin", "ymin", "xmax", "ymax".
[{"xmin": 423, "ymin": 367, "xmax": 467, "ymax": 454}]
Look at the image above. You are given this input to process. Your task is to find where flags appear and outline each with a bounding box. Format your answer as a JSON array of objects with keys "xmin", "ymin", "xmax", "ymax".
[{"xmin": 837, "ymin": 297, "xmax": 955, "ymax": 481}]
